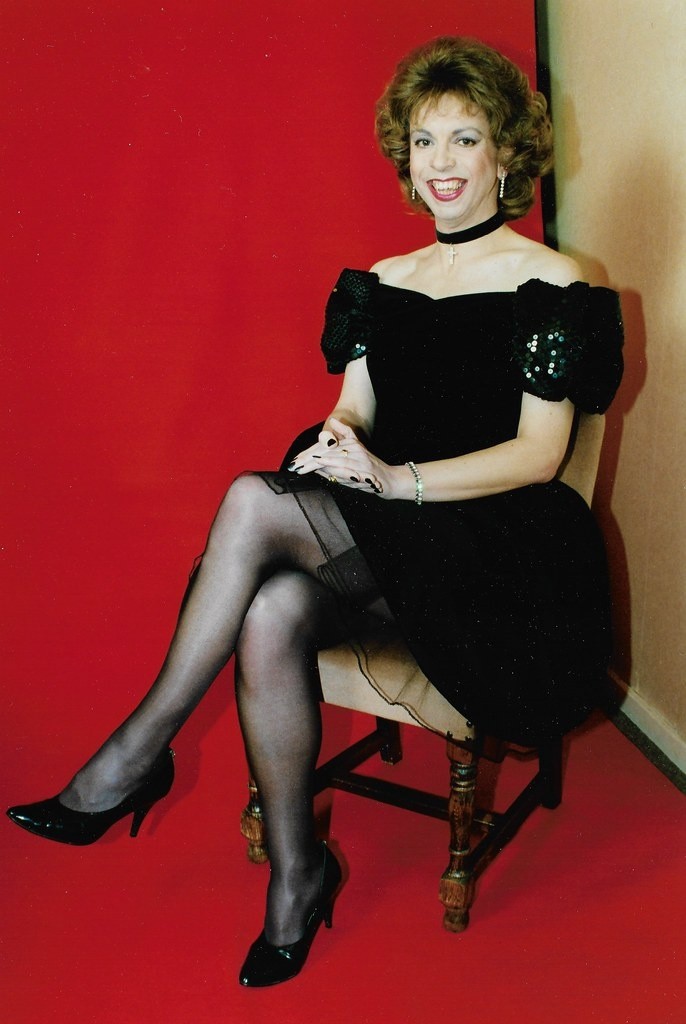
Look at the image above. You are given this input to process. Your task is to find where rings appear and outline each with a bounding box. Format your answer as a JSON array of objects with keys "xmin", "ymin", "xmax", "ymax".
[
  {"xmin": 341, "ymin": 449, "xmax": 349, "ymax": 456},
  {"xmin": 329, "ymin": 474, "xmax": 337, "ymax": 482}
]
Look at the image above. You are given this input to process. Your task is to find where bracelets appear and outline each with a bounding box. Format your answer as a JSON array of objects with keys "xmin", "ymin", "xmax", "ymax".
[{"xmin": 405, "ymin": 462, "xmax": 423, "ymax": 506}]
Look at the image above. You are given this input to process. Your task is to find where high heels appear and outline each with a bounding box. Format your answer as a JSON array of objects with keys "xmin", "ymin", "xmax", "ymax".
[
  {"xmin": 239, "ymin": 840, "xmax": 342, "ymax": 987},
  {"xmin": 6, "ymin": 748, "xmax": 174, "ymax": 845}
]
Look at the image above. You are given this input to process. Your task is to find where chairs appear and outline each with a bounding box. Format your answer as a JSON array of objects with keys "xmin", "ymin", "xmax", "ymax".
[{"xmin": 236, "ymin": 254, "xmax": 611, "ymax": 935}]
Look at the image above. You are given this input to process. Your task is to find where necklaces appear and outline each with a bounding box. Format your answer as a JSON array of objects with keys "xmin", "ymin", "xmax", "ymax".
[{"xmin": 435, "ymin": 209, "xmax": 507, "ymax": 263}]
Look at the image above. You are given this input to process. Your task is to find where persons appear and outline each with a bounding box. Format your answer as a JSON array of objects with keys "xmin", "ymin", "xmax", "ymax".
[{"xmin": 8, "ymin": 34, "xmax": 625, "ymax": 986}]
